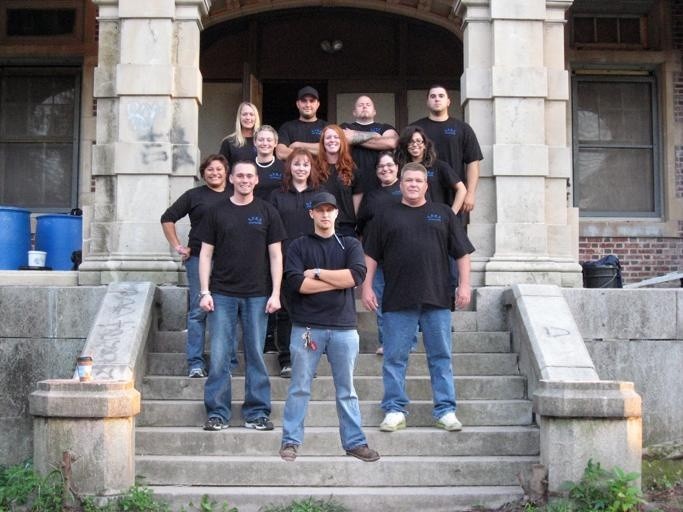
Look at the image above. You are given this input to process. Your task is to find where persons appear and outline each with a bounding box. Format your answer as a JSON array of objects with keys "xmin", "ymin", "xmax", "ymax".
[
  {"xmin": 359, "ymin": 160, "xmax": 476, "ymax": 432},
  {"xmin": 214, "ymin": 81, "xmax": 484, "ymax": 381},
  {"xmin": 279, "ymin": 191, "xmax": 380, "ymax": 463},
  {"xmin": 194, "ymin": 158, "xmax": 289, "ymax": 431},
  {"xmin": 158, "ymin": 151, "xmax": 234, "ymax": 380}
]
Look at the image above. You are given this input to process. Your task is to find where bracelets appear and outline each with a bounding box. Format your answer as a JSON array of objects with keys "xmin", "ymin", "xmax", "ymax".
[
  {"xmin": 197, "ymin": 290, "xmax": 211, "ymax": 298},
  {"xmin": 176, "ymin": 245, "xmax": 184, "ymax": 254}
]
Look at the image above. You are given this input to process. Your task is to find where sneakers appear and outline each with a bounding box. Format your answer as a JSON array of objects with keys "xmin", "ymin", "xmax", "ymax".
[
  {"xmin": 378, "ymin": 411, "xmax": 407, "ymax": 432},
  {"xmin": 280, "ymin": 366, "xmax": 293, "ymax": 378},
  {"xmin": 203, "ymin": 416, "xmax": 231, "ymax": 431},
  {"xmin": 346, "ymin": 443, "xmax": 381, "ymax": 461},
  {"xmin": 263, "ymin": 340, "xmax": 280, "ymax": 354},
  {"xmin": 279, "ymin": 443, "xmax": 299, "ymax": 459},
  {"xmin": 188, "ymin": 367, "xmax": 209, "ymax": 378},
  {"xmin": 244, "ymin": 416, "xmax": 274, "ymax": 431},
  {"xmin": 435, "ymin": 411, "xmax": 462, "ymax": 432}
]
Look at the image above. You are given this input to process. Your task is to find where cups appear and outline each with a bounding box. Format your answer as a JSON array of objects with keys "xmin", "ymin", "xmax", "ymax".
[
  {"xmin": 26, "ymin": 250, "xmax": 48, "ymax": 267},
  {"xmin": 76, "ymin": 355, "xmax": 94, "ymax": 382}
]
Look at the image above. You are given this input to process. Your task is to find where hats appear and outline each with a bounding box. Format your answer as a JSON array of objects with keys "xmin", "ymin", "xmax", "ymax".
[
  {"xmin": 310, "ymin": 192, "xmax": 339, "ymax": 210},
  {"xmin": 296, "ymin": 87, "xmax": 319, "ymax": 101}
]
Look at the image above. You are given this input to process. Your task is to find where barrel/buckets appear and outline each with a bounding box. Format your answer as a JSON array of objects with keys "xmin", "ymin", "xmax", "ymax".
[
  {"xmin": 584, "ymin": 265, "xmax": 622, "ymax": 288},
  {"xmin": 35, "ymin": 215, "xmax": 82, "ymax": 270},
  {"xmin": 0, "ymin": 206, "xmax": 32, "ymax": 270}
]
